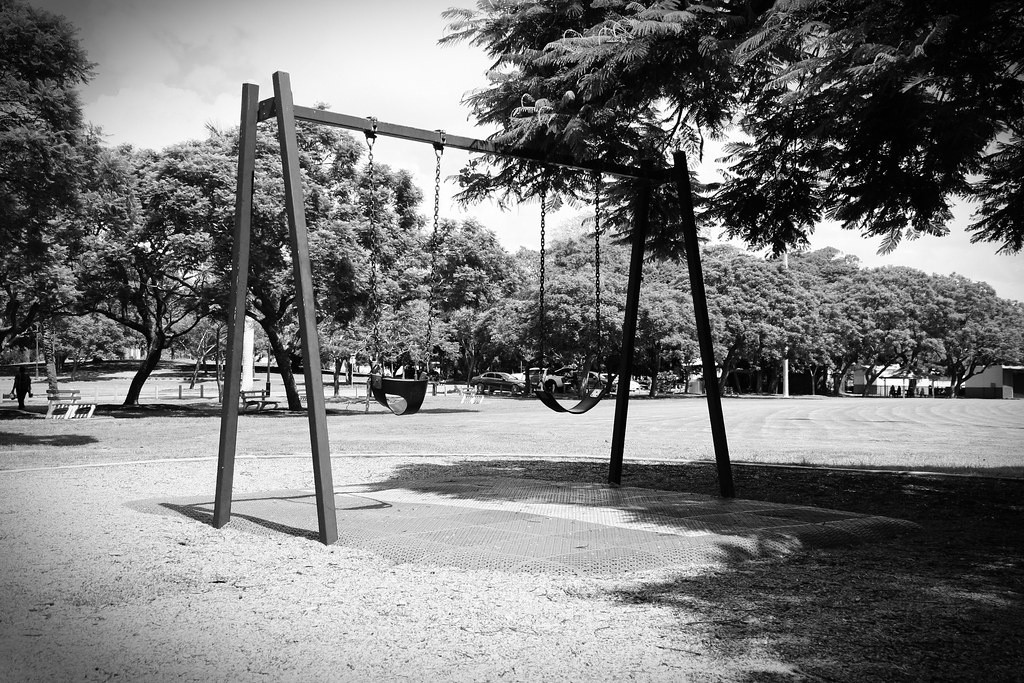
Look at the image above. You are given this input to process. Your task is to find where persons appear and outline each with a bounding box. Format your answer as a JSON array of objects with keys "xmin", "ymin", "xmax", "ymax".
[
  {"xmin": 888, "ymin": 385, "xmax": 901, "ymax": 397},
  {"xmin": 10, "ymin": 365, "xmax": 33, "ymax": 410}
]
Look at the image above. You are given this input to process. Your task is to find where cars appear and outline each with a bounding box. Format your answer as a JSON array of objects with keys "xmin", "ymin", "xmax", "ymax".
[
  {"xmin": 470, "ymin": 372, "xmax": 527, "ymax": 394},
  {"xmin": 513, "ymin": 365, "xmax": 642, "ymax": 394}
]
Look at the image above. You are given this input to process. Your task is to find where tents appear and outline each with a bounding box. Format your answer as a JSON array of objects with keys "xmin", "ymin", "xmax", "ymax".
[{"xmin": 879, "ymin": 371, "xmax": 911, "ymax": 398}]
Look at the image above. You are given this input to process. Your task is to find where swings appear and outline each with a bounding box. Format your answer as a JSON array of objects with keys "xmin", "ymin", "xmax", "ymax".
[
  {"xmin": 531, "ymin": 153, "xmax": 611, "ymax": 415},
  {"xmin": 363, "ymin": 116, "xmax": 448, "ymax": 418}
]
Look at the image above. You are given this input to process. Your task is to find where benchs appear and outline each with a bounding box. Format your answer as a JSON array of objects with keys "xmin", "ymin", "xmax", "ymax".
[
  {"xmin": 46, "ymin": 390, "xmax": 96, "ymax": 420},
  {"xmin": 461, "ymin": 387, "xmax": 484, "ymax": 404},
  {"xmin": 238, "ymin": 389, "xmax": 279, "ymax": 414}
]
